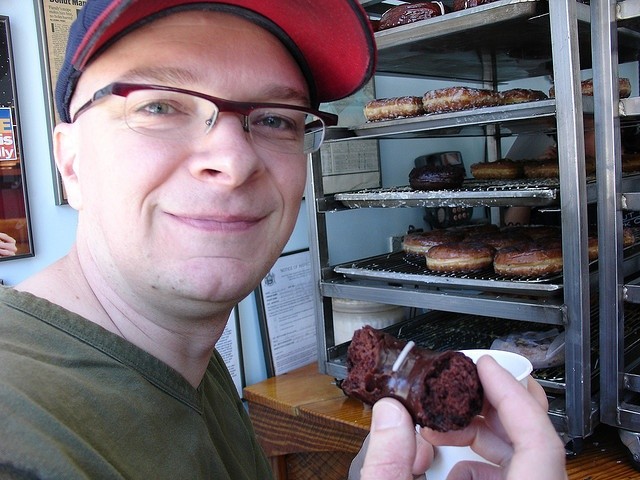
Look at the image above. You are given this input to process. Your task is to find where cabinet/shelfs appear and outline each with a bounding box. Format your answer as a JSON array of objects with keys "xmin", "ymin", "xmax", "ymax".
[
  {"xmin": 582, "ymin": 1, "xmax": 639, "ymax": 432},
  {"xmin": 282, "ymin": 1, "xmax": 599, "ymax": 440}
]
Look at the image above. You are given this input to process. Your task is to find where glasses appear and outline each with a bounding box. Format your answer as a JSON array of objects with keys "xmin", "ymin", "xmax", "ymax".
[{"xmin": 71, "ymin": 82, "xmax": 338, "ymax": 154}]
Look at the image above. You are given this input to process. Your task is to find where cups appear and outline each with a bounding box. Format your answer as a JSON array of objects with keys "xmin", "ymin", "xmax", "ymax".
[{"xmin": 414, "ymin": 349, "xmax": 533, "ymax": 475}]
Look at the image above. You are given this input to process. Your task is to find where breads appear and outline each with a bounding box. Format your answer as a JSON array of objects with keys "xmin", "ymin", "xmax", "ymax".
[
  {"xmin": 364, "ymin": 96, "xmax": 422, "ymax": 119},
  {"xmin": 402, "ymin": 224, "xmax": 634, "ymax": 276},
  {"xmin": 409, "ymin": 164, "xmax": 465, "ymax": 190},
  {"xmin": 503, "ymin": 89, "xmax": 546, "ymax": 104},
  {"xmin": 422, "ymin": 87, "xmax": 501, "ymax": 111},
  {"xmin": 471, "ymin": 151, "xmax": 640, "ymax": 179},
  {"xmin": 380, "ymin": 0, "xmax": 445, "ymax": 27},
  {"xmin": 582, "ymin": 77, "xmax": 631, "ymax": 99}
]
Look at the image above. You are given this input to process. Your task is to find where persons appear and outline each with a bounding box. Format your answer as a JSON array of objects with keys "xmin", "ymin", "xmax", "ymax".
[
  {"xmin": 0, "ymin": 233, "xmax": 18, "ymax": 257},
  {"xmin": 0, "ymin": 0, "xmax": 567, "ymax": 480}
]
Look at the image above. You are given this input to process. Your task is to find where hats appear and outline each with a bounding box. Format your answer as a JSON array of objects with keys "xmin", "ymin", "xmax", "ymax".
[{"xmin": 55, "ymin": 0, "xmax": 378, "ymax": 122}]
